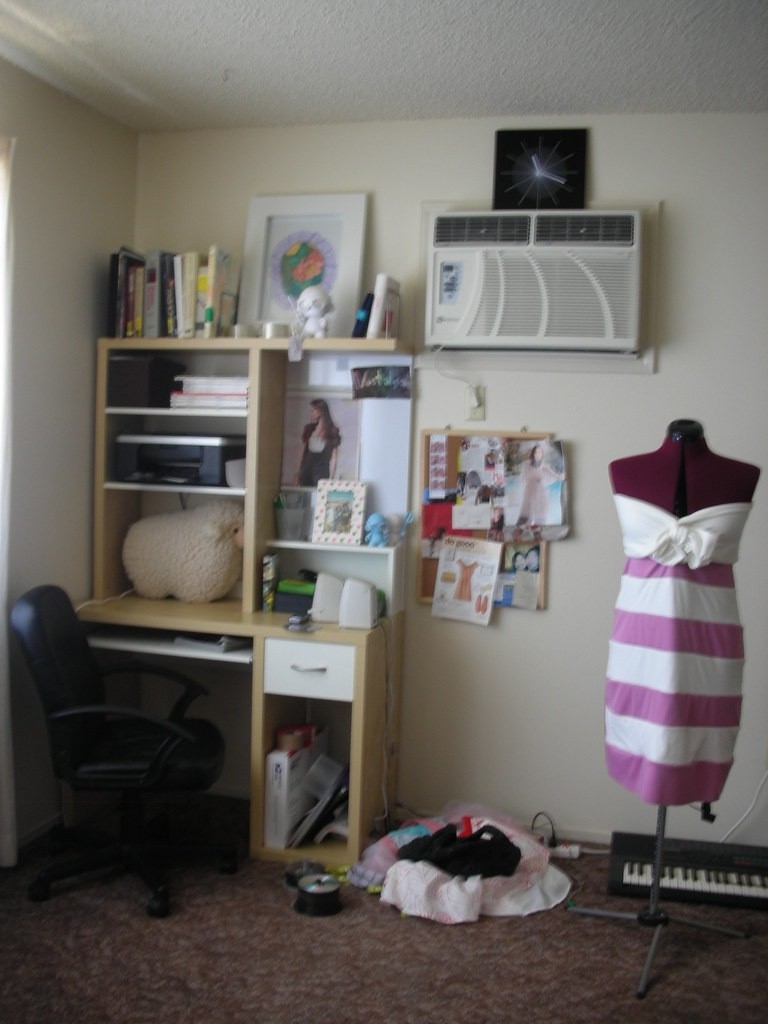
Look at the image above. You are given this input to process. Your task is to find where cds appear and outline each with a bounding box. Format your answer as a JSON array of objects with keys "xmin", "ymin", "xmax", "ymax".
[{"xmin": 298, "ymin": 874, "xmax": 339, "ymax": 893}]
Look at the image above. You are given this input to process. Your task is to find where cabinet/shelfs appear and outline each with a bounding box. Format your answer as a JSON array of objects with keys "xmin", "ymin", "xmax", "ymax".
[{"xmin": 75, "ymin": 337, "xmax": 419, "ymax": 869}]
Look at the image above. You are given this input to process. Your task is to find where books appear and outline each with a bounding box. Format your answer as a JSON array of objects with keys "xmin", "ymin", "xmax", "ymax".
[
  {"xmin": 175, "ymin": 635, "xmax": 249, "ymax": 652},
  {"xmin": 262, "ymin": 553, "xmax": 278, "ymax": 613},
  {"xmin": 108, "ymin": 245, "xmax": 239, "ymax": 338},
  {"xmin": 352, "ymin": 275, "xmax": 401, "ymax": 339},
  {"xmin": 170, "ymin": 375, "xmax": 248, "ymax": 409}
]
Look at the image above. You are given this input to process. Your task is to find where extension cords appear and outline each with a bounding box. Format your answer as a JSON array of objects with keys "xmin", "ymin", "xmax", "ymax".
[{"xmin": 543, "ymin": 843, "xmax": 582, "ymax": 859}]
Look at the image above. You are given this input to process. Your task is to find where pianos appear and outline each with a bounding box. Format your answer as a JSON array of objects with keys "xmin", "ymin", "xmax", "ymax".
[{"xmin": 606, "ymin": 830, "xmax": 768, "ymax": 913}]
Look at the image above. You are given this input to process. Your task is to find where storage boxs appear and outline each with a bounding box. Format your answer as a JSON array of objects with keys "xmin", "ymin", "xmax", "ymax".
[
  {"xmin": 265, "ymin": 723, "xmax": 330, "ymax": 848},
  {"xmin": 113, "ymin": 433, "xmax": 246, "ymax": 484}
]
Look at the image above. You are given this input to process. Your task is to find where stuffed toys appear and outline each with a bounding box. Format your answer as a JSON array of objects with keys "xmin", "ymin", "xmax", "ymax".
[{"xmin": 122, "ymin": 501, "xmax": 244, "ymax": 603}]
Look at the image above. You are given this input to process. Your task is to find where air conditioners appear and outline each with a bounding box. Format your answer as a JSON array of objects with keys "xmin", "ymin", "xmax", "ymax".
[{"xmin": 426, "ymin": 207, "xmax": 641, "ymax": 354}]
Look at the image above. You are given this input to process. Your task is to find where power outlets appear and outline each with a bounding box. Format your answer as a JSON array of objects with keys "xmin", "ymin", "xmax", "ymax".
[{"xmin": 465, "ymin": 385, "xmax": 486, "ymax": 420}]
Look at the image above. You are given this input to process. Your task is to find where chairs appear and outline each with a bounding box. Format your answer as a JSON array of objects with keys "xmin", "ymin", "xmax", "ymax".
[{"xmin": 8, "ymin": 583, "xmax": 242, "ymax": 918}]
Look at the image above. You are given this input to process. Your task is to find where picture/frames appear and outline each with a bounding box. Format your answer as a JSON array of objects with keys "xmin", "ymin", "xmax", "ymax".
[
  {"xmin": 311, "ymin": 478, "xmax": 368, "ymax": 545},
  {"xmin": 235, "ymin": 192, "xmax": 369, "ymax": 337},
  {"xmin": 416, "ymin": 429, "xmax": 551, "ymax": 611},
  {"xmin": 281, "ymin": 389, "xmax": 363, "ymax": 491}
]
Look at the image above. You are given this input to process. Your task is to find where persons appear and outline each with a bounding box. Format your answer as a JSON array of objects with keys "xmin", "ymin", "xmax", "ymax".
[
  {"xmin": 291, "ymin": 398, "xmax": 341, "ymax": 488},
  {"xmin": 333, "ymin": 503, "xmax": 352, "ymax": 532},
  {"xmin": 297, "ymin": 287, "xmax": 334, "ymax": 339},
  {"xmin": 458, "ymin": 443, "xmax": 564, "ymax": 542},
  {"xmin": 512, "ymin": 546, "xmax": 540, "ymax": 574},
  {"xmin": 604, "ymin": 419, "xmax": 761, "ymax": 806}
]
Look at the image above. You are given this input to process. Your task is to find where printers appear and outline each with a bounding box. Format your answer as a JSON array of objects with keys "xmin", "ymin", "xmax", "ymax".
[{"xmin": 114, "ymin": 435, "xmax": 246, "ymax": 488}]
